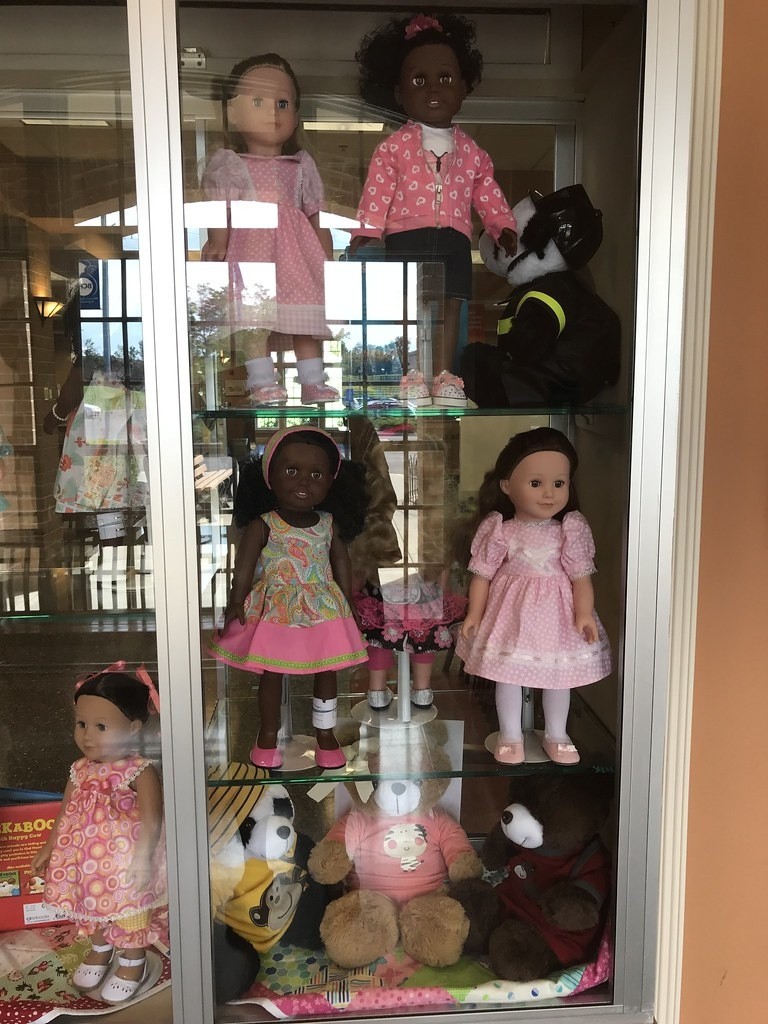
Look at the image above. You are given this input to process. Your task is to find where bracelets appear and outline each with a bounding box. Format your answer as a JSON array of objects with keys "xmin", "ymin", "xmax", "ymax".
[{"xmin": 52, "ymin": 402, "xmax": 70, "ymax": 421}]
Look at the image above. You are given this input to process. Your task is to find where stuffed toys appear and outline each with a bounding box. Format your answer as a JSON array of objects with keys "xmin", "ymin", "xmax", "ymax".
[
  {"xmin": 452, "ymin": 184, "xmax": 620, "ymax": 407},
  {"xmin": 308, "ymin": 717, "xmax": 489, "ymax": 968},
  {"xmin": 206, "ymin": 785, "xmax": 344, "ymax": 1004},
  {"xmin": 448, "ymin": 746, "xmax": 612, "ymax": 982}
]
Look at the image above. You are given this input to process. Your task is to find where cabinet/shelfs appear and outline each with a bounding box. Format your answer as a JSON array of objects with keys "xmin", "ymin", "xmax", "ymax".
[{"xmin": 196, "ymin": 385, "xmax": 622, "ymax": 789}]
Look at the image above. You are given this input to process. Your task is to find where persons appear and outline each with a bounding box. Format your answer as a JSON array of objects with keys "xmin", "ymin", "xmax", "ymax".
[
  {"xmin": 454, "ymin": 427, "xmax": 613, "ymax": 766},
  {"xmin": 352, "ymin": 426, "xmax": 468, "ymax": 711},
  {"xmin": 44, "ymin": 267, "xmax": 154, "ymax": 584},
  {"xmin": 200, "ymin": 52, "xmax": 341, "ymax": 408},
  {"xmin": 206, "ymin": 426, "xmax": 368, "ymax": 770},
  {"xmin": 30, "ymin": 660, "xmax": 163, "ymax": 1004},
  {"xmin": 348, "ymin": 10, "xmax": 517, "ymax": 408},
  {"xmin": 227, "ymin": 321, "xmax": 401, "ymax": 793}
]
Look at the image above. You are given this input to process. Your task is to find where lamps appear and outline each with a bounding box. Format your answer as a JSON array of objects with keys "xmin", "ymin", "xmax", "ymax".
[{"xmin": 32, "ymin": 296, "xmax": 67, "ymax": 328}]
